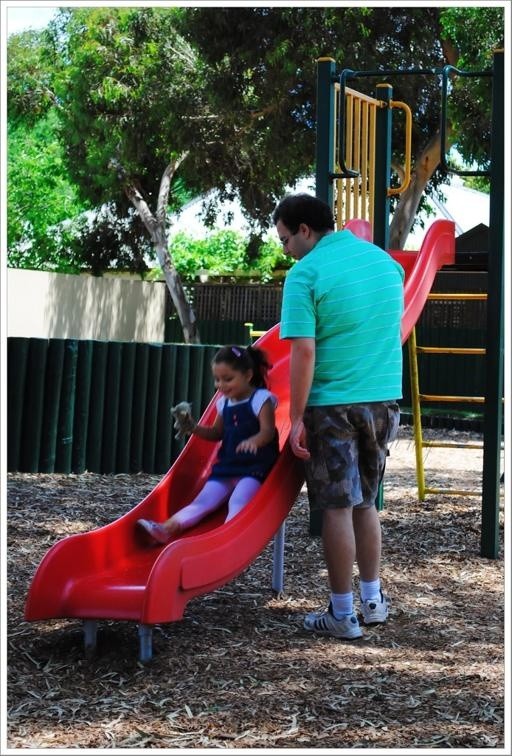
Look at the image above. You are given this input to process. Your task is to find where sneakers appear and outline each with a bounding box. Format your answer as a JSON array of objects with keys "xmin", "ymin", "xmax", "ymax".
[
  {"xmin": 357, "ymin": 592, "xmax": 392, "ymax": 624},
  {"xmin": 298, "ymin": 605, "xmax": 364, "ymax": 641},
  {"xmin": 138, "ymin": 513, "xmax": 174, "ymax": 544}
]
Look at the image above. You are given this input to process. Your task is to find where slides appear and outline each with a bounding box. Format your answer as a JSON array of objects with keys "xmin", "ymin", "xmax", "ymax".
[{"xmin": 23, "ymin": 217, "xmax": 456, "ymax": 626}]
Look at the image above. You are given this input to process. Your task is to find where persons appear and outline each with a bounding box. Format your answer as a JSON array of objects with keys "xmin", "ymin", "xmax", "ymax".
[
  {"xmin": 273, "ymin": 195, "xmax": 406, "ymax": 639},
  {"xmin": 136, "ymin": 342, "xmax": 279, "ymax": 545}
]
